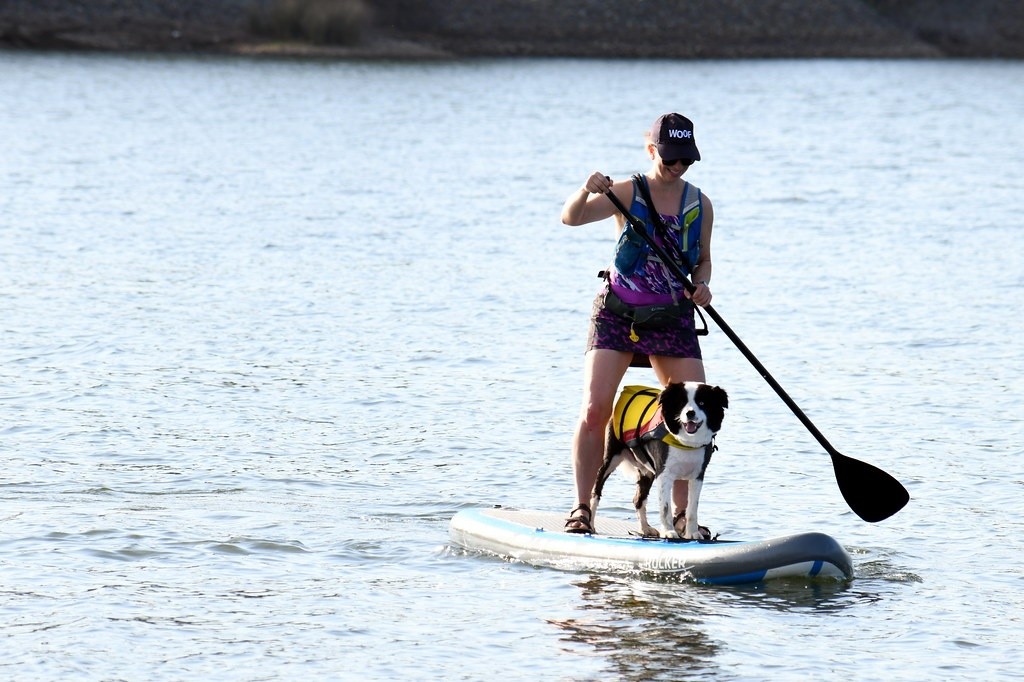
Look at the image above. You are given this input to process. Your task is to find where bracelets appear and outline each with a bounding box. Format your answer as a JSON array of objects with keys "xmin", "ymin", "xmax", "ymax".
[{"xmin": 695, "ymin": 281, "xmax": 708, "ymax": 288}]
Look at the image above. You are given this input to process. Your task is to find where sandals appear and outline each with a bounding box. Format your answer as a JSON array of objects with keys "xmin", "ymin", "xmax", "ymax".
[
  {"xmin": 565, "ymin": 503, "xmax": 594, "ymax": 533},
  {"xmin": 673, "ymin": 510, "xmax": 712, "ymax": 540}
]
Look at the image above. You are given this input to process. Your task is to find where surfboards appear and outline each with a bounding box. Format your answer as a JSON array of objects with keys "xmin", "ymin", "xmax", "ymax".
[{"xmin": 447, "ymin": 505, "xmax": 854, "ymax": 585}]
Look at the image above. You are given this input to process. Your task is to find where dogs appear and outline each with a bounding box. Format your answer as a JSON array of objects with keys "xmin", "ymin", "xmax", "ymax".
[{"xmin": 590, "ymin": 381, "xmax": 729, "ymax": 540}]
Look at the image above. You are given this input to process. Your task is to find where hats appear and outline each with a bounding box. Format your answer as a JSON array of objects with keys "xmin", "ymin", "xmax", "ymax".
[{"xmin": 650, "ymin": 114, "xmax": 701, "ymax": 161}]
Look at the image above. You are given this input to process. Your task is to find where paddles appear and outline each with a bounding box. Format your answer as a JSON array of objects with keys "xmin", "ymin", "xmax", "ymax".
[{"xmin": 592, "ymin": 175, "xmax": 911, "ymax": 524}]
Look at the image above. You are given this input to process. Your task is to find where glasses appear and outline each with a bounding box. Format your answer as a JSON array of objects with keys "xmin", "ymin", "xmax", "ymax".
[{"xmin": 662, "ymin": 157, "xmax": 696, "ymax": 167}]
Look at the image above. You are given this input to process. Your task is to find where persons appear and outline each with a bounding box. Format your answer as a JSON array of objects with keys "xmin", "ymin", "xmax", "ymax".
[{"xmin": 562, "ymin": 112, "xmax": 714, "ymax": 540}]
